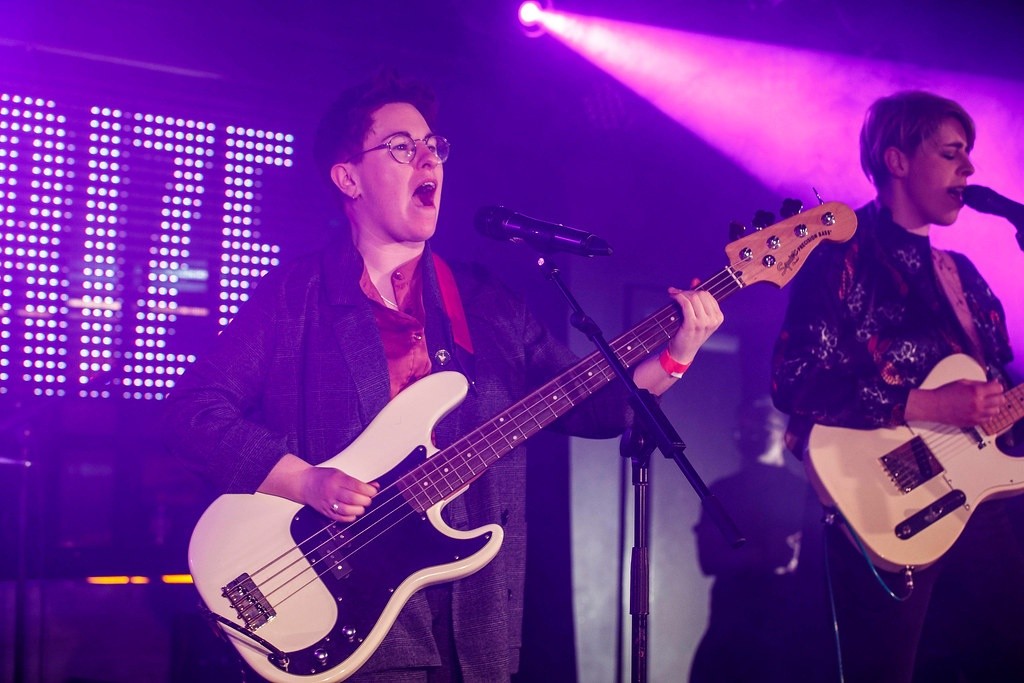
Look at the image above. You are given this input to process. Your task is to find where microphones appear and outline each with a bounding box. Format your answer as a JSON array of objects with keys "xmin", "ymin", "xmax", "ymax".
[
  {"xmin": 962, "ymin": 184, "xmax": 1024, "ymax": 220},
  {"xmin": 473, "ymin": 205, "xmax": 614, "ymax": 258}
]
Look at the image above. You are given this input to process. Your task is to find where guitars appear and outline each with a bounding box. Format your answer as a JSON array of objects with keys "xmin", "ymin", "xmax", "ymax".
[
  {"xmin": 186, "ymin": 185, "xmax": 857, "ymax": 683},
  {"xmin": 804, "ymin": 352, "xmax": 1024, "ymax": 574}
]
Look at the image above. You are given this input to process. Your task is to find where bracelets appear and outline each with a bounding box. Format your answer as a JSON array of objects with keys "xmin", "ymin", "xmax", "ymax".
[{"xmin": 658, "ymin": 348, "xmax": 691, "ymax": 379}]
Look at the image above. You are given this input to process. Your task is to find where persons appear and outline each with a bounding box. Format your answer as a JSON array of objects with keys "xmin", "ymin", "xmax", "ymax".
[
  {"xmin": 689, "ymin": 392, "xmax": 818, "ymax": 680},
  {"xmin": 156, "ymin": 73, "xmax": 726, "ymax": 682},
  {"xmin": 770, "ymin": 94, "xmax": 1022, "ymax": 639}
]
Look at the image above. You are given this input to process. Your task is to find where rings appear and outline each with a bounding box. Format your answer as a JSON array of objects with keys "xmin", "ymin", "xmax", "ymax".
[{"xmin": 330, "ymin": 502, "xmax": 338, "ymax": 512}]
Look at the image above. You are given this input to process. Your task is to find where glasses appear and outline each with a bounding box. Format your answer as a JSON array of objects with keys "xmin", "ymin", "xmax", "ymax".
[{"xmin": 344, "ymin": 133, "xmax": 451, "ymax": 165}]
